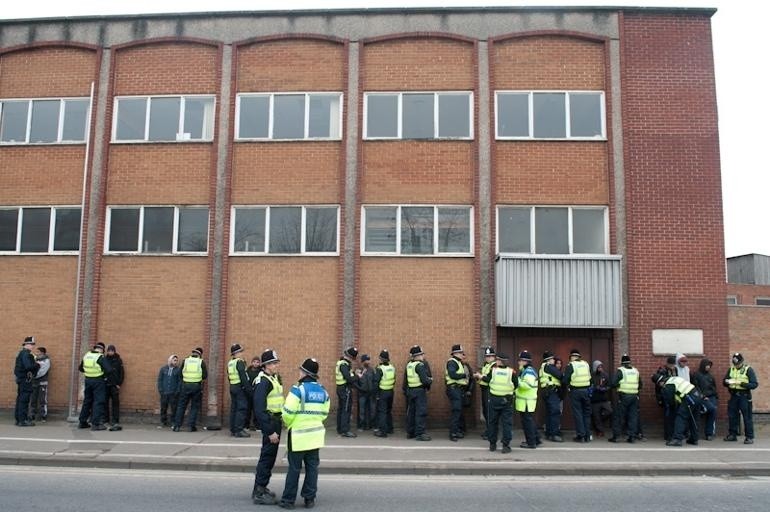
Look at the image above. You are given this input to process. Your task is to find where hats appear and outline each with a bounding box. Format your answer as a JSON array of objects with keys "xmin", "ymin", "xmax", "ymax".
[{"xmin": 230, "ymin": 344, "xmax": 244, "ymax": 356}]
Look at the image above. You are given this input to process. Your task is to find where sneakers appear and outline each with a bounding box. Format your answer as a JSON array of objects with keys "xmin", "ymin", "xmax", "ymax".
[
  {"xmin": 450, "ymin": 433, "xmax": 542, "ymax": 453},
  {"xmin": 15, "ymin": 415, "xmax": 46, "ymax": 426},
  {"xmin": 157, "ymin": 422, "xmax": 197, "ymax": 432},
  {"xmin": 253, "ymin": 489, "xmax": 277, "ymax": 504},
  {"xmin": 336, "ymin": 426, "xmax": 431, "ymax": 441},
  {"xmin": 545, "ymin": 433, "xmax": 648, "ymax": 443},
  {"xmin": 230, "ymin": 427, "xmax": 262, "ymax": 437},
  {"xmin": 663, "ymin": 434, "xmax": 754, "ymax": 446},
  {"xmin": 305, "ymin": 499, "xmax": 315, "ymax": 508},
  {"xmin": 278, "ymin": 501, "xmax": 294, "ymax": 509},
  {"xmin": 78, "ymin": 419, "xmax": 121, "ymax": 431}
]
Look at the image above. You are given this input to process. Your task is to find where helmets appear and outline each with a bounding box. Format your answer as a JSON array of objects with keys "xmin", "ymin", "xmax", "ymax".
[
  {"xmin": 651, "ymin": 373, "xmax": 664, "ymax": 385},
  {"xmin": 93, "ymin": 342, "xmax": 105, "ymax": 353},
  {"xmin": 450, "ymin": 344, "xmax": 583, "ymax": 362},
  {"xmin": 298, "ymin": 358, "xmax": 320, "ymax": 379},
  {"xmin": 260, "ymin": 349, "xmax": 280, "ymax": 368},
  {"xmin": 732, "ymin": 353, "xmax": 744, "ymax": 365},
  {"xmin": 344, "ymin": 345, "xmax": 423, "ymax": 362},
  {"xmin": 22, "ymin": 336, "xmax": 36, "ymax": 345},
  {"xmin": 192, "ymin": 347, "xmax": 202, "ymax": 358},
  {"xmin": 621, "ymin": 355, "xmax": 631, "ymax": 365}
]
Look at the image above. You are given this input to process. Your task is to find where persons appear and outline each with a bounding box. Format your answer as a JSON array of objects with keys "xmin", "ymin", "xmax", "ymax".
[
  {"xmin": 538, "ymin": 349, "xmax": 648, "ymax": 443},
  {"xmin": 157, "ymin": 347, "xmax": 208, "ymax": 433},
  {"xmin": 78, "ymin": 342, "xmax": 125, "ymax": 430},
  {"xmin": 402, "ymin": 345, "xmax": 433, "ymax": 441},
  {"xmin": 228, "ymin": 344, "xmax": 261, "ymax": 438},
  {"xmin": 335, "ymin": 346, "xmax": 396, "ymax": 437},
  {"xmin": 249, "ymin": 349, "xmax": 284, "ymax": 506},
  {"xmin": 723, "ymin": 352, "xmax": 759, "ymax": 444},
  {"xmin": 445, "ymin": 344, "xmax": 542, "ymax": 455},
  {"xmin": 277, "ymin": 357, "xmax": 332, "ymax": 511},
  {"xmin": 651, "ymin": 351, "xmax": 719, "ymax": 446},
  {"xmin": 13, "ymin": 333, "xmax": 51, "ymax": 426}
]
